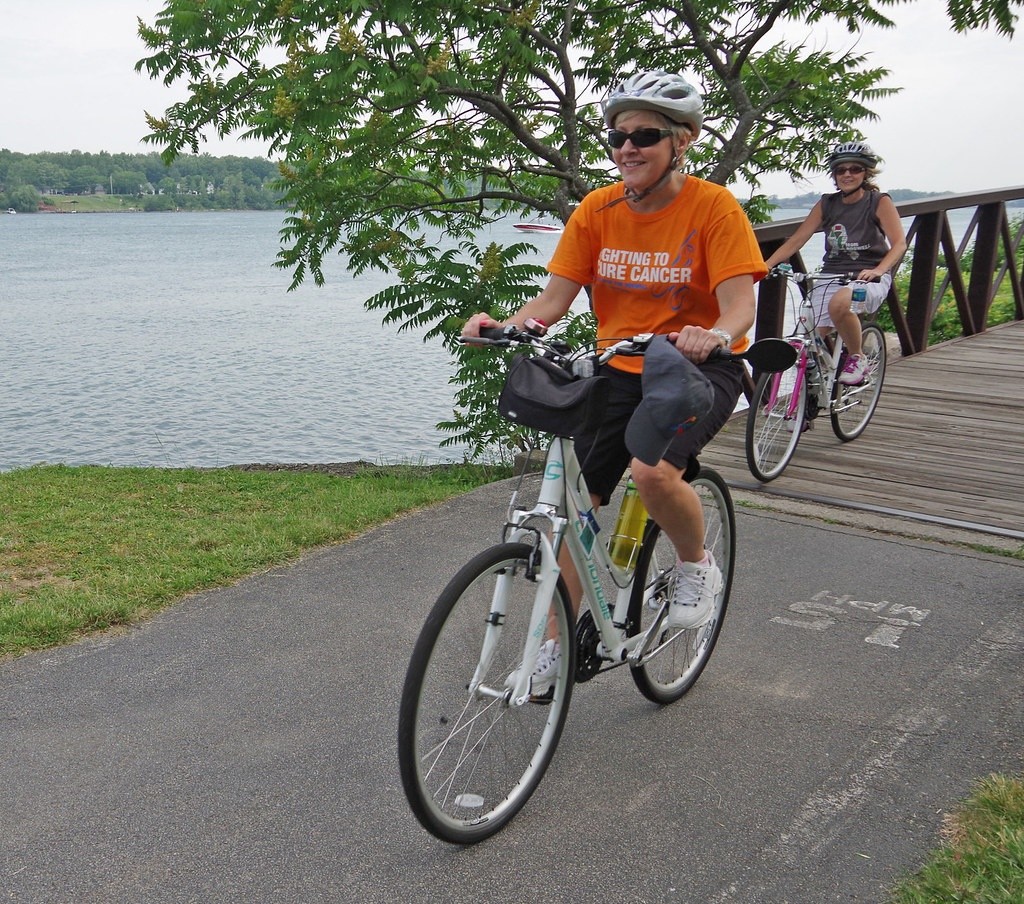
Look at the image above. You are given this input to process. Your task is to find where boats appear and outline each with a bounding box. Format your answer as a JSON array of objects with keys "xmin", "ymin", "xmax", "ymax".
[
  {"xmin": 512, "ymin": 223, "xmax": 562, "ymax": 233},
  {"xmin": 2, "ymin": 208, "xmax": 16, "ymax": 214}
]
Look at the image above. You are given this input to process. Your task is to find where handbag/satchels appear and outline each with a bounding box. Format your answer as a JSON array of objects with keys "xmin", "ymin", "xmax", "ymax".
[{"xmin": 497, "ymin": 353, "xmax": 612, "ymax": 440}]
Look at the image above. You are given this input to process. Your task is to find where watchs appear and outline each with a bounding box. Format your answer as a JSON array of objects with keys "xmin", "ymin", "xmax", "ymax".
[{"xmin": 709, "ymin": 327, "xmax": 732, "ymax": 347}]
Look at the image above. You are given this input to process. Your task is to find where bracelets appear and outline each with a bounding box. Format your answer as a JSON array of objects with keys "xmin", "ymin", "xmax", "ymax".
[{"xmin": 765, "ymin": 261, "xmax": 773, "ymax": 270}]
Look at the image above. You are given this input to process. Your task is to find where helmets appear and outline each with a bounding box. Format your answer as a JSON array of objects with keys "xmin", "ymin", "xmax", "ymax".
[
  {"xmin": 827, "ymin": 142, "xmax": 878, "ymax": 169},
  {"xmin": 600, "ymin": 69, "xmax": 703, "ymax": 141}
]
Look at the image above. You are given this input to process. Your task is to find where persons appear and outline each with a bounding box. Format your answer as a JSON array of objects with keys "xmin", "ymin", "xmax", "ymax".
[
  {"xmin": 462, "ymin": 74, "xmax": 770, "ymax": 701},
  {"xmin": 764, "ymin": 141, "xmax": 907, "ymax": 433}
]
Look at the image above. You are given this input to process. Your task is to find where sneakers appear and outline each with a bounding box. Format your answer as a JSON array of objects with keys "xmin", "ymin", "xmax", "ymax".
[
  {"xmin": 838, "ymin": 354, "xmax": 870, "ymax": 385},
  {"xmin": 787, "ymin": 414, "xmax": 808, "ymax": 433},
  {"xmin": 505, "ymin": 639, "xmax": 562, "ymax": 697},
  {"xmin": 664, "ymin": 549, "xmax": 723, "ymax": 630}
]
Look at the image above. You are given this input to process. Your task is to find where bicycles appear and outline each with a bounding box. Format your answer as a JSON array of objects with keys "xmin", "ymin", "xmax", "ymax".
[
  {"xmin": 398, "ymin": 317, "xmax": 798, "ymax": 845},
  {"xmin": 745, "ymin": 261, "xmax": 887, "ymax": 482}
]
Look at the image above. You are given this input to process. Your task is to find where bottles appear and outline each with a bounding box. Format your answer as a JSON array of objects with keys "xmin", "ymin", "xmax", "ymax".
[
  {"xmin": 849, "ymin": 280, "xmax": 867, "ymax": 314},
  {"xmin": 806, "ymin": 358, "xmax": 822, "ymax": 395},
  {"xmin": 608, "ymin": 474, "xmax": 650, "ymax": 570}
]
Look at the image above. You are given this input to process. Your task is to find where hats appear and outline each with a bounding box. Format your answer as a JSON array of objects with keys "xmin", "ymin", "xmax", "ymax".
[{"xmin": 624, "ymin": 333, "xmax": 715, "ymax": 467}]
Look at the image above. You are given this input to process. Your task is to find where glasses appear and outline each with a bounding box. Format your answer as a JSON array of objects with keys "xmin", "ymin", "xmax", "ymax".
[
  {"xmin": 832, "ymin": 165, "xmax": 865, "ymax": 174},
  {"xmin": 605, "ymin": 128, "xmax": 673, "ymax": 147}
]
